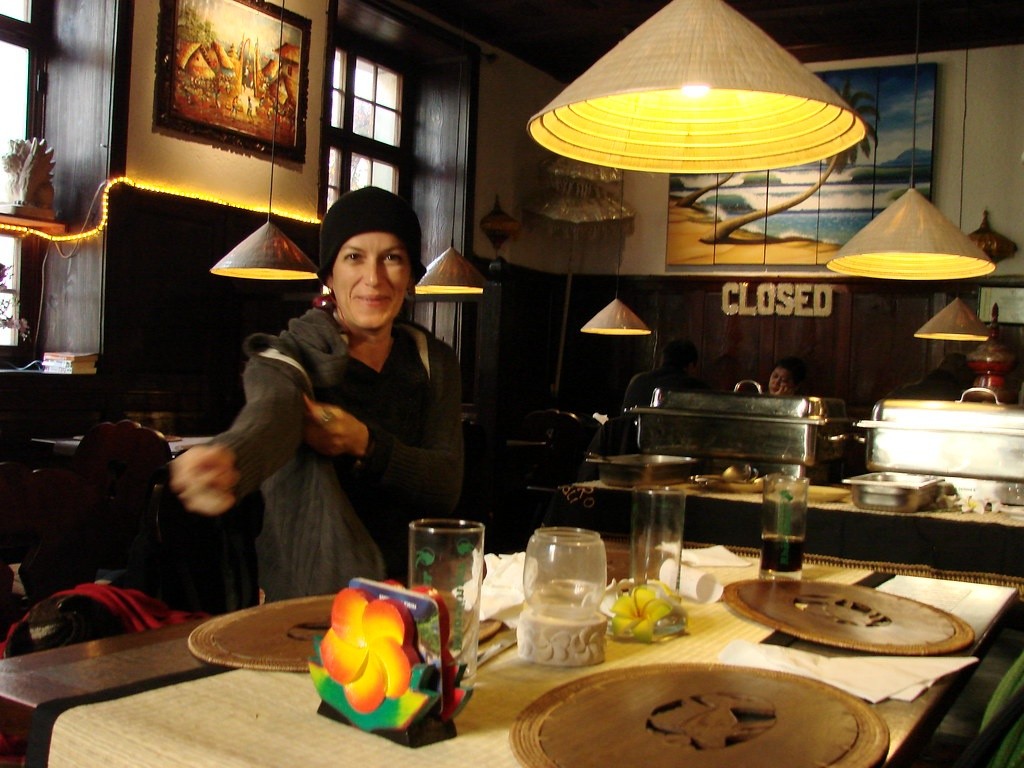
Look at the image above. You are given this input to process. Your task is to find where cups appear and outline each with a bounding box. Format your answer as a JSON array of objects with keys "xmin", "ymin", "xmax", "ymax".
[
  {"xmin": 756, "ymin": 473, "xmax": 809, "ymax": 581},
  {"xmin": 408, "ymin": 519, "xmax": 486, "ymax": 691},
  {"xmin": 522, "ymin": 526, "xmax": 608, "ymax": 622},
  {"xmin": 629, "ymin": 489, "xmax": 686, "ymax": 596}
]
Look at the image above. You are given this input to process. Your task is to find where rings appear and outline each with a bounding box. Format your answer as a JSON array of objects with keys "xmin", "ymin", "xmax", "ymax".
[{"xmin": 323, "ymin": 414, "xmax": 331, "ymax": 425}]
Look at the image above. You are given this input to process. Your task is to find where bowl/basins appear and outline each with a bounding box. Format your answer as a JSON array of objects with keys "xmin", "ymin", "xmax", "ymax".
[
  {"xmin": 586, "ymin": 453, "xmax": 697, "ymax": 488},
  {"xmin": 841, "ymin": 471, "xmax": 945, "ymax": 512}
]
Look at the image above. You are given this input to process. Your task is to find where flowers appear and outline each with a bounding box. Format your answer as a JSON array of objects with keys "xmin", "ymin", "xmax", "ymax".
[
  {"xmin": 582, "ymin": 579, "xmax": 673, "ymax": 642},
  {"xmin": 306, "ymin": 587, "xmax": 439, "ymax": 732},
  {"xmin": 452, "ymin": 551, "xmax": 538, "ymax": 629}
]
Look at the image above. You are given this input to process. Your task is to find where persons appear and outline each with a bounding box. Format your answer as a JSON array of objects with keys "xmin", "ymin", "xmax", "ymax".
[
  {"xmin": 768, "ymin": 355, "xmax": 806, "ymax": 394},
  {"xmin": 886, "ymin": 352, "xmax": 969, "ymax": 400},
  {"xmin": 170, "ymin": 187, "xmax": 464, "ymax": 603},
  {"xmin": 618, "ymin": 338, "xmax": 712, "ymax": 417}
]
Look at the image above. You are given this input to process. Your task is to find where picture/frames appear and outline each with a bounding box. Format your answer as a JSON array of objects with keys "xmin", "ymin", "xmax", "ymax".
[{"xmin": 154, "ymin": 0, "xmax": 312, "ymax": 163}]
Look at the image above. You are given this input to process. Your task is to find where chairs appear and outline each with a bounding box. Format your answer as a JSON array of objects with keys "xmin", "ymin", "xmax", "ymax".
[
  {"xmin": 520, "ymin": 411, "xmax": 581, "ymax": 544},
  {"xmin": 0, "ymin": 420, "xmax": 172, "ymax": 657}
]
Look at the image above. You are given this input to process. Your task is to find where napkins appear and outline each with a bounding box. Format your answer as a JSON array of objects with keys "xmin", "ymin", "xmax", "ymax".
[
  {"xmin": 718, "ymin": 638, "xmax": 982, "ymax": 704},
  {"xmin": 657, "ymin": 543, "xmax": 753, "ymax": 567}
]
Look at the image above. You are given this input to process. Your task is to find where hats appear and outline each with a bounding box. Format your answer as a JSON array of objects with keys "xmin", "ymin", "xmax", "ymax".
[{"xmin": 319, "ymin": 186, "xmax": 423, "ymax": 285}]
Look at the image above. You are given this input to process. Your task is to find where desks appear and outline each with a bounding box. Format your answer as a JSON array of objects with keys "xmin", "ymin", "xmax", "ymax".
[
  {"xmin": 572, "ymin": 473, "xmax": 1024, "ymax": 577},
  {"xmin": 29, "ymin": 435, "xmax": 228, "ymax": 615},
  {"xmin": 0, "ymin": 538, "xmax": 1020, "ymax": 768}
]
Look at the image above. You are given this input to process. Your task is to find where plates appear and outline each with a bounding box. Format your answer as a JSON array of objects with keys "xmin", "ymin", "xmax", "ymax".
[
  {"xmin": 690, "ymin": 475, "xmax": 765, "ymax": 491},
  {"xmin": 807, "ymin": 485, "xmax": 849, "ymax": 501}
]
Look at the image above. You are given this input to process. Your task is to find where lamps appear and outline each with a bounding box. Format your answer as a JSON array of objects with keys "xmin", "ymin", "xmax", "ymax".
[
  {"xmin": 209, "ymin": 1, "xmax": 320, "ymax": 280},
  {"xmin": 913, "ymin": 49, "xmax": 991, "ymax": 341},
  {"xmin": 580, "ymin": 170, "xmax": 651, "ymax": 336},
  {"xmin": 527, "ymin": 0, "xmax": 867, "ymax": 174},
  {"xmin": 415, "ymin": 28, "xmax": 488, "ymax": 295},
  {"xmin": 827, "ymin": 0, "xmax": 997, "ymax": 281}
]
